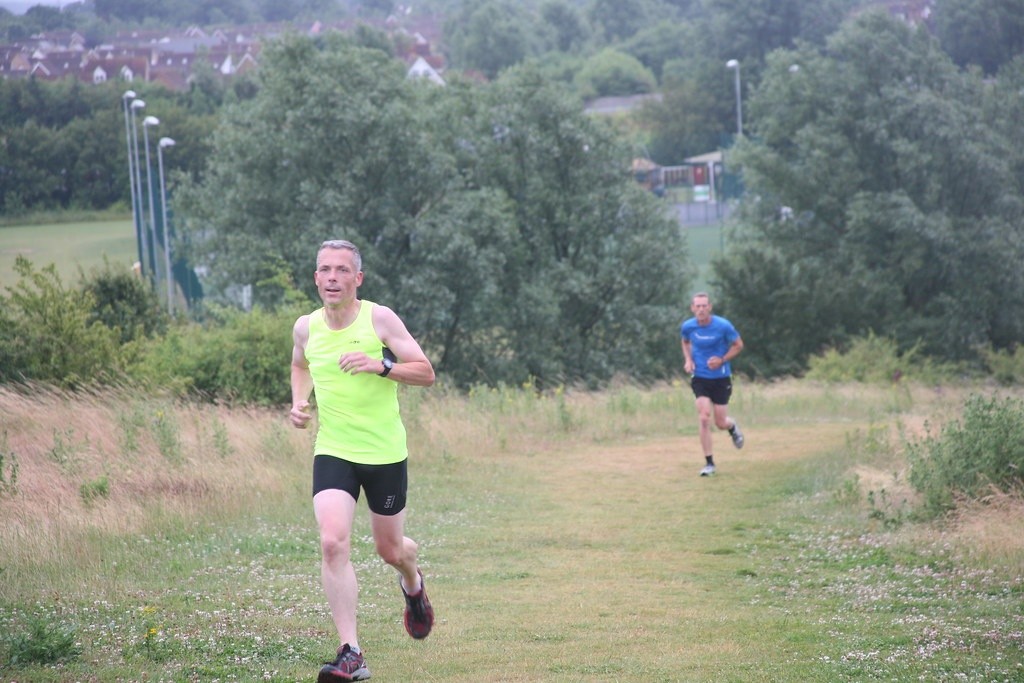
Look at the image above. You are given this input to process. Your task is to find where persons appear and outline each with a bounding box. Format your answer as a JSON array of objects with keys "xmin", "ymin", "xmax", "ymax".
[
  {"xmin": 680, "ymin": 293, "xmax": 744, "ymax": 478},
  {"xmin": 288, "ymin": 240, "xmax": 436, "ymax": 681}
]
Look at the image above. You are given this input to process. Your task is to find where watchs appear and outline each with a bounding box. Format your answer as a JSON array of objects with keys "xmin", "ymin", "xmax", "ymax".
[{"xmin": 379, "ymin": 358, "xmax": 392, "ymax": 377}]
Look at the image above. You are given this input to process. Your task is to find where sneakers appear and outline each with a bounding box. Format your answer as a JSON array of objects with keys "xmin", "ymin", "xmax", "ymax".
[
  {"xmin": 318, "ymin": 643, "xmax": 371, "ymax": 683},
  {"xmin": 399, "ymin": 566, "xmax": 434, "ymax": 639},
  {"xmin": 700, "ymin": 463, "xmax": 715, "ymax": 476},
  {"xmin": 729, "ymin": 421, "xmax": 744, "ymax": 448}
]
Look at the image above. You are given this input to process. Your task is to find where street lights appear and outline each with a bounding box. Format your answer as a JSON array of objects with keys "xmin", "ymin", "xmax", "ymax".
[
  {"xmin": 157, "ymin": 137, "xmax": 177, "ymax": 315},
  {"xmin": 130, "ymin": 99, "xmax": 146, "ymax": 263},
  {"xmin": 723, "ymin": 56, "xmax": 743, "ymax": 135},
  {"xmin": 121, "ymin": 89, "xmax": 137, "ymax": 238},
  {"xmin": 142, "ymin": 115, "xmax": 161, "ymax": 283}
]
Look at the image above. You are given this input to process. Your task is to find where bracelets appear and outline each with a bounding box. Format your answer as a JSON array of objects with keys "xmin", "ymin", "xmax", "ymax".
[{"xmin": 721, "ymin": 357, "xmax": 724, "ymax": 364}]
